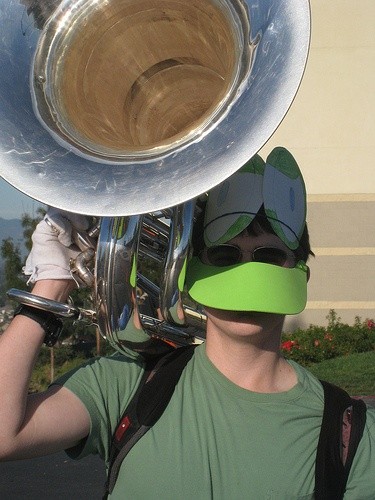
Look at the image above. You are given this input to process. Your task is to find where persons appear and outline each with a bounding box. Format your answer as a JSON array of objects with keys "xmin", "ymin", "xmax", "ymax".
[{"xmin": 0, "ymin": 147, "xmax": 374, "ymax": 500}]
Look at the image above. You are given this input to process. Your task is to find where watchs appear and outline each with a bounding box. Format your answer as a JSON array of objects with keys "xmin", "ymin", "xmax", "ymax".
[{"xmin": 10, "ymin": 305, "xmax": 68, "ymax": 345}]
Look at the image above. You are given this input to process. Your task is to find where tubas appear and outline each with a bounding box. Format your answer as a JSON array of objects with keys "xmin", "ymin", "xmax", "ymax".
[{"xmin": 1, "ymin": 0, "xmax": 312, "ymax": 362}]
